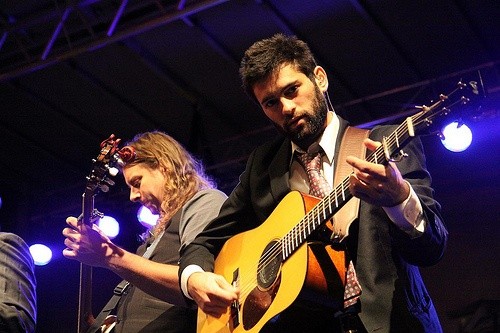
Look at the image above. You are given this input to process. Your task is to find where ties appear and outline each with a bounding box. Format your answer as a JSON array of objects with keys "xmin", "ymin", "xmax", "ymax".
[{"xmin": 296, "ymin": 150, "xmax": 362, "ymax": 310}]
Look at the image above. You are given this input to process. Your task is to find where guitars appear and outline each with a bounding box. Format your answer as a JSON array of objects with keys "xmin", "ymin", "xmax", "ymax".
[
  {"xmin": 196, "ymin": 70, "xmax": 489, "ymax": 333},
  {"xmin": 77, "ymin": 134, "xmax": 134, "ymax": 333}
]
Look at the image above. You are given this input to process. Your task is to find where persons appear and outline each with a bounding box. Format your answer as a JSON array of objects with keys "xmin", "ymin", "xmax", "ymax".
[
  {"xmin": 0, "ymin": 232, "xmax": 38, "ymax": 333},
  {"xmin": 62, "ymin": 131, "xmax": 228, "ymax": 333},
  {"xmin": 177, "ymin": 33, "xmax": 450, "ymax": 333}
]
